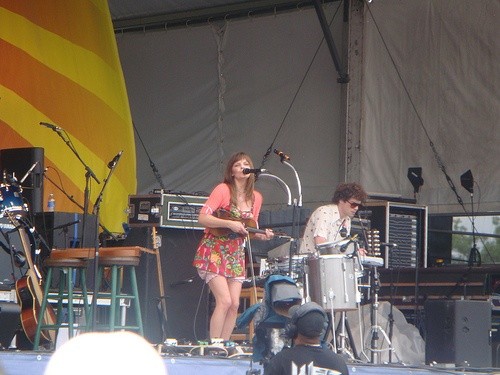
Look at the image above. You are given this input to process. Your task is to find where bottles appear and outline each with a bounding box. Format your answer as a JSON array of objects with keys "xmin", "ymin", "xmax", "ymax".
[{"xmin": 47, "ymin": 193, "xmax": 55, "ymax": 211}]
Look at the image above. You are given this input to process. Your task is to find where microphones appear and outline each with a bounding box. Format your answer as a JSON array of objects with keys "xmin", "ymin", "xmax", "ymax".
[
  {"xmin": 274, "ymin": 148, "xmax": 290, "ymax": 160},
  {"xmin": 243, "ymin": 168, "xmax": 267, "ymax": 174},
  {"xmin": 108, "ymin": 152, "xmax": 122, "ymax": 169},
  {"xmin": 40, "ymin": 122, "xmax": 62, "ymax": 130},
  {"xmin": 172, "ymin": 279, "xmax": 193, "ymax": 286},
  {"xmin": 380, "ymin": 243, "xmax": 397, "ymax": 247},
  {"xmin": 340, "ymin": 220, "xmax": 347, "ymax": 237}
]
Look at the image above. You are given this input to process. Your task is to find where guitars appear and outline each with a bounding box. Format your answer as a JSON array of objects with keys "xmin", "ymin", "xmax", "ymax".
[
  {"xmin": 4, "ymin": 208, "xmax": 58, "ymax": 343},
  {"xmin": 211, "ymin": 207, "xmax": 293, "ymax": 241}
]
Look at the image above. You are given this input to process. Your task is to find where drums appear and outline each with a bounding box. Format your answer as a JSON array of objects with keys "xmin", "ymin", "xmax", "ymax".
[{"xmin": 0, "ymin": 174, "xmax": 28, "ymax": 229}]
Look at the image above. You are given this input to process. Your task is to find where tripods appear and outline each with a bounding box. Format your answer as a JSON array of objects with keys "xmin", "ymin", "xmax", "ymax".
[{"xmin": 356, "ymin": 265, "xmax": 409, "ymax": 367}]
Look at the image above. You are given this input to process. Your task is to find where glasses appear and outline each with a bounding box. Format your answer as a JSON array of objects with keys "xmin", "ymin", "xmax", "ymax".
[{"xmin": 346, "ymin": 200, "xmax": 361, "ymax": 208}]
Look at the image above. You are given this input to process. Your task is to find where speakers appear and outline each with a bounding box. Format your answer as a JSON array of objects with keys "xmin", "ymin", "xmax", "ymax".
[
  {"xmin": 127, "ymin": 228, "xmax": 208, "ymax": 346},
  {"xmin": 35, "ymin": 211, "xmax": 100, "ymax": 294},
  {"xmin": 424, "ymin": 300, "xmax": 493, "ymax": 372},
  {"xmin": 0, "ymin": 217, "xmax": 37, "ymax": 291},
  {"xmin": 0, "ymin": 147, "xmax": 44, "ymax": 216}
]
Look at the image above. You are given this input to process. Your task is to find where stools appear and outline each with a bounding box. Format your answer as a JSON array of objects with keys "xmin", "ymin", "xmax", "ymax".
[
  {"xmin": 86, "ymin": 255, "xmax": 145, "ymax": 339},
  {"xmin": 230, "ymin": 285, "xmax": 265, "ymax": 344},
  {"xmin": 33, "ymin": 256, "xmax": 90, "ymax": 350}
]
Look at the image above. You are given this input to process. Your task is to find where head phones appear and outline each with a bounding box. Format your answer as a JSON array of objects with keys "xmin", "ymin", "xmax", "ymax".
[{"xmin": 285, "ymin": 301, "xmax": 329, "ymax": 337}]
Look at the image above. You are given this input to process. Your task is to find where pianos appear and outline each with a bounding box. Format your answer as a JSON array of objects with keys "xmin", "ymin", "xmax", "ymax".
[{"xmin": 293, "ymin": 253, "xmax": 389, "ymax": 267}]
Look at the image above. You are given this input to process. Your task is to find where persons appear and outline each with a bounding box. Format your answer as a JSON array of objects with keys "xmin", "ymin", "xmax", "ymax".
[
  {"xmin": 193, "ymin": 151, "xmax": 274, "ymax": 347},
  {"xmin": 265, "ymin": 302, "xmax": 348, "ymax": 374},
  {"xmin": 299, "ymin": 183, "xmax": 368, "ymax": 256}
]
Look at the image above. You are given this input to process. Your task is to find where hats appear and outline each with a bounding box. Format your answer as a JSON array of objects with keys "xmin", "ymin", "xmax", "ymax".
[{"xmin": 288, "ymin": 304, "xmax": 325, "ymax": 336}]
